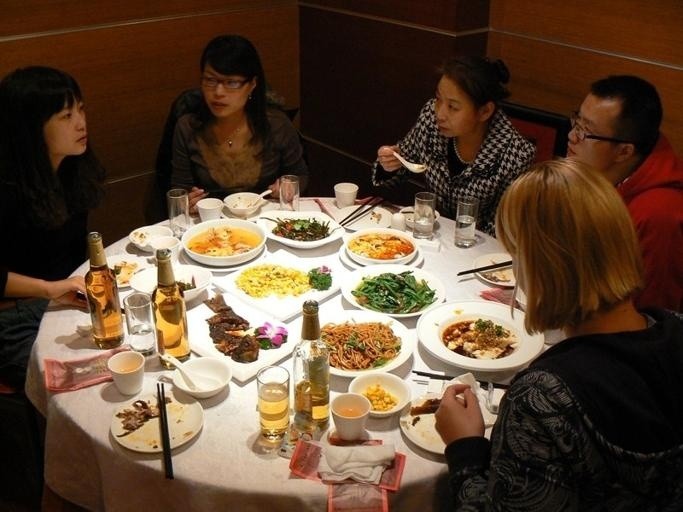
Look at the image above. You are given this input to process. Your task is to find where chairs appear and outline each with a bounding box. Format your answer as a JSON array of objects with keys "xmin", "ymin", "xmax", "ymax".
[
  {"xmin": 144, "ymin": 89, "xmax": 283, "ymax": 222},
  {"xmin": 494, "ymin": 97, "xmax": 570, "ymax": 168},
  {"xmin": 0, "ymin": 385, "xmax": 36, "ymax": 465}
]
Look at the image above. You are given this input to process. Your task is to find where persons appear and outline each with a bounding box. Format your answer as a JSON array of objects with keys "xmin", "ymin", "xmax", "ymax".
[
  {"xmin": 370, "ymin": 54, "xmax": 537, "ymax": 241},
  {"xmin": 562, "ymin": 75, "xmax": 682, "ymax": 316},
  {"xmin": 0, "ymin": 64, "xmax": 115, "ymax": 403},
  {"xmin": 434, "ymin": 153, "xmax": 681, "ymax": 512},
  {"xmin": 168, "ymin": 33, "xmax": 311, "ymax": 219}
]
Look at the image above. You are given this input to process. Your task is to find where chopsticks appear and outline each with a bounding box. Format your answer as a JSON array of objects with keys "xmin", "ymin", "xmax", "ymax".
[
  {"xmin": 457, "ymin": 260, "xmax": 513, "ymax": 276},
  {"xmin": 156, "ymin": 383, "xmax": 174, "ymax": 480},
  {"xmin": 411, "ymin": 370, "xmax": 512, "ymax": 390},
  {"xmin": 338, "ymin": 194, "xmax": 383, "ymax": 228}
]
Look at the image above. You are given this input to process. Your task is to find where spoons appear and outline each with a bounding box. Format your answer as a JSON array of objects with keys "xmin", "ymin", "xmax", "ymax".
[
  {"xmin": 243, "ymin": 188, "xmax": 272, "ymax": 211},
  {"xmin": 161, "ymin": 353, "xmax": 224, "ymax": 390},
  {"xmin": 381, "ymin": 147, "xmax": 427, "ymax": 173}
]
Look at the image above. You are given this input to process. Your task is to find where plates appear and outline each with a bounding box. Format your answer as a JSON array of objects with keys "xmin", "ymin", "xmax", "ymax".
[
  {"xmin": 129, "ymin": 263, "xmax": 213, "ymax": 303},
  {"xmin": 213, "ymin": 249, "xmax": 340, "ymax": 322},
  {"xmin": 341, "ymin": 264, "xmax": 447, "ymax": 319},
  {"xmin": 104, "ymin": 254, "xmax": 148, "ymax": 289},
  {"xmin": 398, "ymin": 392, "xmax": 493, "ymax": 456},
  {"xmin": 110, "ymin": 389, "xmax": 204, "ymax": 454},
  {"xmin": 182, "ymin": 217, "xmax": 266, "ymax": 267},
  {"xmin": 255, "ymin": 210, "xmax": 345, "ymax": 250},
  {"xmin": 338, "ymin": 228, "xmax": 424, "ymax": 270},
  {"xmin": 473, "ymin": 252, "xmax": 518, "ymax": 287},
  {"xmin": 185, "ymin": 291, "xmax": 300, "ymax": 384},
  {"xmin": 287, "ymin": 309, "xmax": 415, "ymax": 378},
  {"xmin": 326, "ymin": 428, "xmax": 371, "ymax": 446},
  {"xmin": 417, "ymin": 300, "xmax": 545, "ymax": 373}
]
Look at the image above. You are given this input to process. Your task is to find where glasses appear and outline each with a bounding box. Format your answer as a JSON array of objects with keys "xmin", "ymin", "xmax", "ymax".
[
  {"xmin": 201, "ymin": 77, "xmax": 251, "ymax": 89},
  {"xmin": 568, "ymin": 111, "xmax": 631, "ymax": 144}
]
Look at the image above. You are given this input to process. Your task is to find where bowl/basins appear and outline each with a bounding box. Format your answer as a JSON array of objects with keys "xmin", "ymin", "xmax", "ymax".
[
  {"xmin": 150, "ymin": 238, "xmax": 182, "ymax": 252},
  {"xmin": 173, "ymin": 357, "xmax": 233, "ymax": 399},
  {"xmin": 347, "ymin": 371, "xmax": 413, "ymax": 419},
  {"xmin": 225, "ymin": 191, "xmax": 263, "ymax": 215},
  {"xmin": 130, "ymin": 225, "xmax": 172, "ymax": 252}
]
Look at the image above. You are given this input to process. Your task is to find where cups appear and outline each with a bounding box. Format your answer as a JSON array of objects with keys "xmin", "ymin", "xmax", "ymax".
[
  {"xmin": 454, "ymin": 193, "xmax": 480, "ymax": 246},
  {"xmin": 330, "ymin": 393, "xmax": 371, "ymax": 440},
  {"xmin": 333, "ymin": 183, "xmax": 359, "ymax": 210},
  {"xmin": 123, "ymin": 292, "xmax": 157, "ymax": 354},
  {"xmin": 486, "ymin": 374, "xmax": 513, "ymax": 414},
  {"xmin": 108, "ymin": 351, "xmax": 145, "ymax": 396},
  {"xmin": 166, "ymin": 186, "xmax": 190, "ymax": 238},
  {"xmin": 197, "ymin": 198, "xmax": 224, "ymax": 221},
  {"xmin": 279, "ymin": 174, "xmax": 300, "ymax": 212},
  {"xmin": 257, "ymin": 365, "xmax": 289, "ymax": 443},
  {"xmin": 412, "ymin": 191, "xmax": 435, "ymax": 239}
]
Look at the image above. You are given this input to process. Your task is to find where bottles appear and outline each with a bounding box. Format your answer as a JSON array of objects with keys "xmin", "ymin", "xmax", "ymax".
[
  {"xmin": 83, "ymin": 231, "xmax": 126, "ymax": 350},
  {"xmin": 151, "ymin": 248, "xmax": 191, "ymax": 361},
  {"xmin": 291, "ymin": 297, "xmax": 330, "ymax": 436}
]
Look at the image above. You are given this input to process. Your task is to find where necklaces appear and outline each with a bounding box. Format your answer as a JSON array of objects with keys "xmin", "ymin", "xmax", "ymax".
[
  {"xmin": 210, "ymin": 116, "xmax": 244, "ymax": 148},
  {"xmin": 450, "ymin": 137, "xmax": 480, "ymax": 166}
]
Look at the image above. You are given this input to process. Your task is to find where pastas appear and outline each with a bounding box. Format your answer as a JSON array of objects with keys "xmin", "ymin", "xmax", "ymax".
[{"xmin": 321, "ymin": 322, "xmax": 401, "ymax": 371}]
